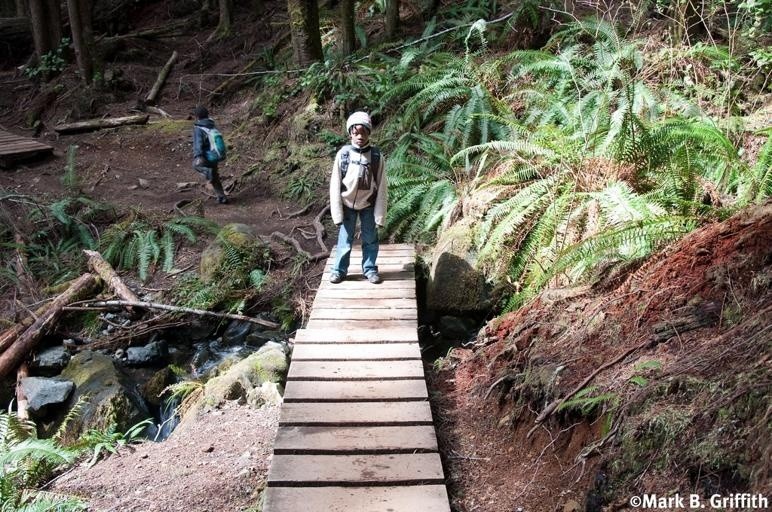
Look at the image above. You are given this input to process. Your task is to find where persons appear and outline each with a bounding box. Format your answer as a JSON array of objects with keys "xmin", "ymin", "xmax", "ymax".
[
  {"xmin": 330, "ymin": 111, "xmax": 387, "ymax": 283},
  {"xmin": 192, "ymin": 105, "xmax": 228, "ymax": 204}
]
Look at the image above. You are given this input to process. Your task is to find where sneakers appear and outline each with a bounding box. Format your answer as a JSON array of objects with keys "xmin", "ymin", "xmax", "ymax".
[
  {"xmin": 215, "ymin": 194, "xmax": 227, "ymax": 204},
  {"xmin": 363, "ymin": 269, "xmax": 382, "ymax": 284},
  {"xmin": 330, "ymin": 269, "xmax": 348, "ymax": 283},
  {"xmin": 204, "ymin": 167, "xmax": 213, "ymax": 184}
]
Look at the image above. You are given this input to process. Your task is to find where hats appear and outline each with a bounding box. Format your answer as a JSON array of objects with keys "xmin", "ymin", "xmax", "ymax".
[{"xmin": 347, "ymin": 111, "xmax": 372, "ymax": 134}]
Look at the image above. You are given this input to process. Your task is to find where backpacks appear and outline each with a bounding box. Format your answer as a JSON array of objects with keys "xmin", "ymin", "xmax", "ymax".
[{"xmin": 205, "ymin": 127, "xmax": 226, "ymax": 163}]
